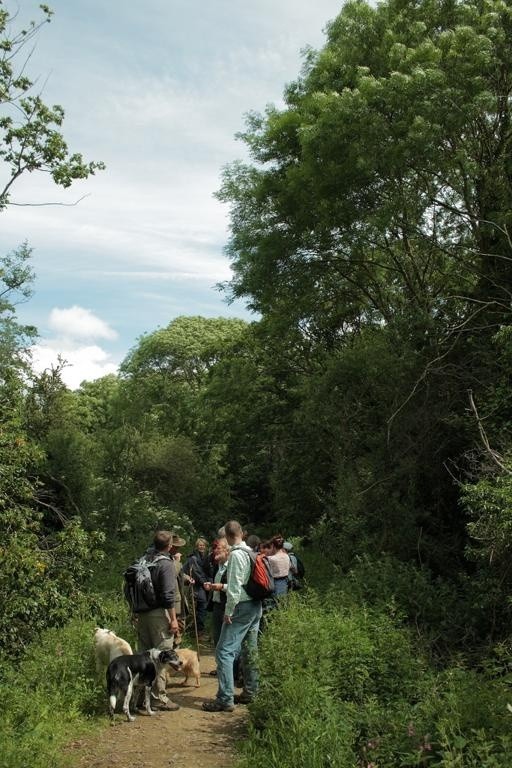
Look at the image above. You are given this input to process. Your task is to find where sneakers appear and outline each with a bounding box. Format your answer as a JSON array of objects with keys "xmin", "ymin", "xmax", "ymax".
[
  {"xmin": 233, "ymin": 694, "xmax": 251, "ymax": 704},
  {"xmin": 198, "ymin": 634, "xmax": 212, "ymax": 642},
  {"xmin": 201, "ymin": 700, "xmax": 236, "ymax": 712},
  {"xmin": 152, "ymin": 699, "xmax": 179, "ymax": 711}
]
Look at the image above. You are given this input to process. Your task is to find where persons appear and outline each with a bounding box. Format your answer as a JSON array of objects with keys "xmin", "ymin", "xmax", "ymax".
[
  {"xmin": 183, "ymin": 526, "xmax": 305, "ymax": 642},
  {"xmin": 169, "ymin": 533, "xmax": 196, "ymax": 649},
  {"xmin": 202, "ymin": 521, "xmax": 263, "ymax": 713},
  {"xmin": 131, "ymin": 531, "xmax": 180, "ymax": 711},
  {"xmin": 203, "ymin": 537, "xmax": 239, "ymax": 680}
]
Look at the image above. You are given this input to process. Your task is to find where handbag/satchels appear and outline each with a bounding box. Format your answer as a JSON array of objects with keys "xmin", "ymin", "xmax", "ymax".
[{"xmin": 289, "ymin": 566, "xmax": 302, "ymax": 591}]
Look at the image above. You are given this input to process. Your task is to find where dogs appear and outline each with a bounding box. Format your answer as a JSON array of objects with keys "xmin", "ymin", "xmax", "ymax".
[
  {"xmin": 106, "ymin": 648, "xmax": 182, "ymax": 726},
  {"xmin": 118, "ymin": 648, "xmax": 161, "ymax": 710},
  {"xmin": 167, "ymin": 649, "xmax": 200, "ymax": 688},
  {"xmin": 93, "ymin": 628, "xmax": 134, "ymax": 701}
]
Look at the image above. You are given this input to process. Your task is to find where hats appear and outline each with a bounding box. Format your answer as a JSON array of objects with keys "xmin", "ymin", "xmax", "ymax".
[
  {"xmin": 172, "ymin": 535, "xmax": 186, "ymax": 547},
  {"xmin": 282, "ymin": 542, "xmax": 293, "ymax": 551}
]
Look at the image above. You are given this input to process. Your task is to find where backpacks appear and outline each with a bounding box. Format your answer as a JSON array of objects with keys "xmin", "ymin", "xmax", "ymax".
[
  {"xmin": 293, "ymin": 555, "xmax": 305, "ymax": 579},
  {"xmin": 230, "ymin": 547, "xmax": 275, "ymax": 601},
  {"xmin": 182, "ymin": 558, "xmax": 193, "ymax": 586},
  {"xmin": 123, "ymin": 555, "xmax": 172, "ymax": 613},
  {"xmin": 203, "ymin": 552, "xmax": 216, "ymax": 578}
]
